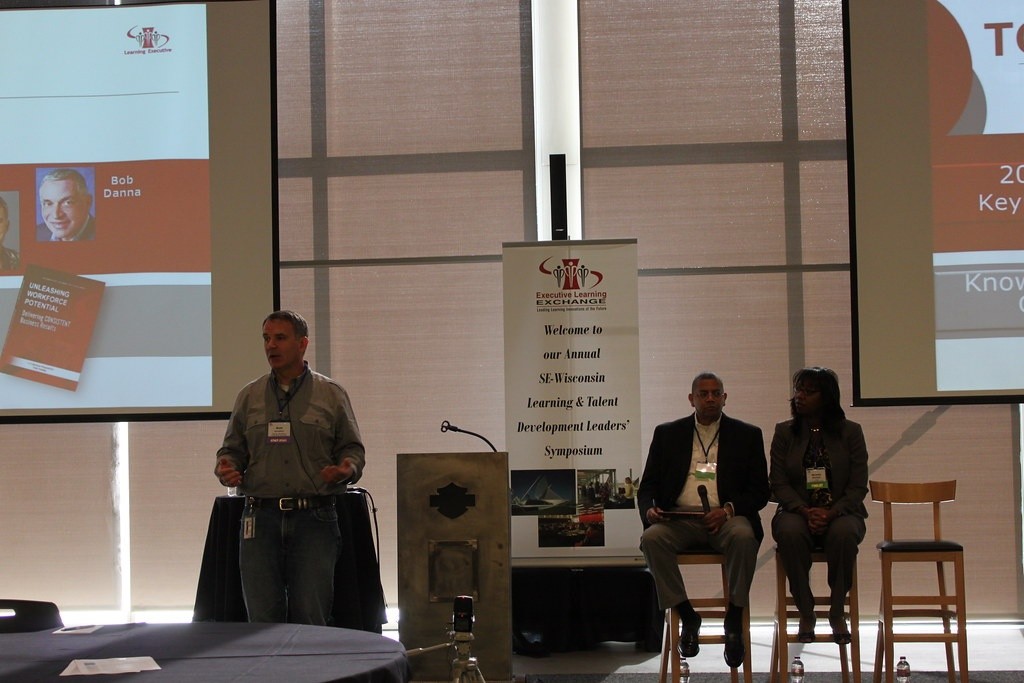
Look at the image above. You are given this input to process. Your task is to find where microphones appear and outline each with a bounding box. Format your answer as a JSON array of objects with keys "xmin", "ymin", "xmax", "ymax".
[{"xmin": 698, "ymin": 485, "xmax": 711, "ymax": 515}]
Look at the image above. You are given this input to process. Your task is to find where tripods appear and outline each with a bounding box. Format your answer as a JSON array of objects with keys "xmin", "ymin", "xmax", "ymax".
[{"xmin": 405, "ymin": 631, "xmax": 486, "ymax": 683}]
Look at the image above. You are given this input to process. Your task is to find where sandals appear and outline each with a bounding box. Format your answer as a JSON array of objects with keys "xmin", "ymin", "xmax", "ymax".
[
  {"xmin": 798, "ymin": 613, "xmax": 816, "ymax": 643},
  {"xmin": 828, "ymin": 611, "xmax": 851, "ymax": 644}
]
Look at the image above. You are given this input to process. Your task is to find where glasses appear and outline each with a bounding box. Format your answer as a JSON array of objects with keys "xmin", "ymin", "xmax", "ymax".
[
  {"xmin": 692, "ymin": 391, "xmax": 725, "ymax": 401},
  {"xmin": 793, "ymin": 384, "xmax": 822, "ymax": 395}
]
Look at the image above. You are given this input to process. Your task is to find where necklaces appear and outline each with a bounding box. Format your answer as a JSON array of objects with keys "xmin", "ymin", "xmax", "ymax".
[{"xmin": 810, "ymin": 428, "xmax": 821, "ymax": 432}]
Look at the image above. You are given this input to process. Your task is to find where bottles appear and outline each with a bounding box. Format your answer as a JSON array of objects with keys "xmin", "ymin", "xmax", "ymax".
[
  {"xmin": 897, "ymin": 656, "xmax": 911, "ymax": 683},
  {"xmin": 789, "ymin": 656, "xmax": 805, "ymax": 683},
  {"xmin": 679, "ymin": 658, "xmax": 690, "ymax": 683}
]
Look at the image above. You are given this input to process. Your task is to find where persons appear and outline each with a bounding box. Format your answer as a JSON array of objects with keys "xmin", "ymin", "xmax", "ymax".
[
  {"xmin": 36, "ymin": 169, "xmax": 95, "ymax": 242},
  {"xmin": 623, "ymin": 477, "xmax": 635, "ymax": 510},
  {"xmin": 214, "ymin": 310, "xmax": 365, "ymax": 626},
  {"xmin": 769, "ymin": 366, "xmax": 869, "ymax": 645},
  {"xmin": 0, "ymin": 196, "xmax": 19, "ymax": 271},
  {"xmin": 539, "ymin": 520, "xmax": 605, "ymax": 546},
  {"xmin": 637, "ymin": 373, "xmax": 770, "ymax": 667},
  {"xmin": 581, "ymin": 481, "xmax": 610, "ymax": 506}
]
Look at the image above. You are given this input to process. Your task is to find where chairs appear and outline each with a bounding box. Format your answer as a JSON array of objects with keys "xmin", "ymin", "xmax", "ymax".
[{"xmin": 0, "ymin": 598, "xmax": 64, "ymax": 634}]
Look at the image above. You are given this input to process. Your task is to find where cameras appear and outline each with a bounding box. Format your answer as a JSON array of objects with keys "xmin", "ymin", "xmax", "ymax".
[{"xmin": 453, "ymin": 595, "xmax": 473, "ymax": 632}]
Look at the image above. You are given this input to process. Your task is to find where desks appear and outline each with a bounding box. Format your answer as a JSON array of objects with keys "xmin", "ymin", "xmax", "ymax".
[{"xmin": 0, "ymin": 623, "xmax": 409, "ymax": 683}]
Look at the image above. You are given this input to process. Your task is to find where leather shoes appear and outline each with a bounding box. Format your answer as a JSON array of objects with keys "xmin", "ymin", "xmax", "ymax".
[
  {"xmin": 677, "ymin": 613, "xmax": 702, "ymax": 657},
  {"xmin": 724, "ymin": 621, "xmax": 745, "ymax": 668}
]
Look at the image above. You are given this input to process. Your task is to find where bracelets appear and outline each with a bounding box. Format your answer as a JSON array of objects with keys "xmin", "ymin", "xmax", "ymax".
[{"xmin": 722, "ymin": 508, "xmax": 731, "ymax": 521}]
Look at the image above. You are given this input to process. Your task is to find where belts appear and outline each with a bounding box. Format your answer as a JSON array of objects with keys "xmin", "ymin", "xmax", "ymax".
[{"xmin": 245, "ymin": 495, "xmax": 334, "ymax": 510}]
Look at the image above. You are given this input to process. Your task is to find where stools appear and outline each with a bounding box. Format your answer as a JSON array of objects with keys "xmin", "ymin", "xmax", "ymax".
[
  {"xmin": 658, "ymin": 554, "xmax": 752, "ymax": 683},
  {"xmin": 866, "ymin": 478, "xmax": 969, "ymax": 683},
  {"xmin": 768, "ymin": 482, "xmax": 862, "ymax": 683}
]
